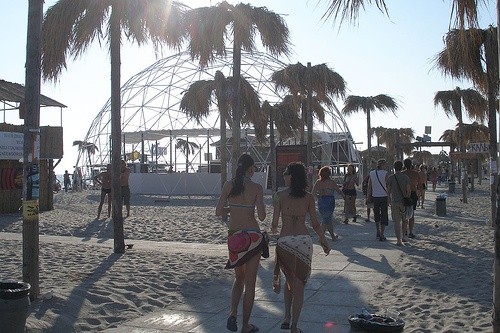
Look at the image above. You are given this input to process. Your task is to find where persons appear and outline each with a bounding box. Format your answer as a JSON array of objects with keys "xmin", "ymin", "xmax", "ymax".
[
  {"xmin": 365, "ymin": 159, "xmax": 390, "ymax": 241},
  {"xmin": 120, "ymin": 160, "xmax": 130, "ymax": 218},
  {"xmin": 432, "ymin": 164, "xmax": 452, "ymax": 183},
  {"xmin": 342, "ymin": 164, "xmax": 360, "ymax": 224},
  {"xmin": 310, "ymin": 165, "xmax": 346, "ymax": 241},
  {"xmin": 388, "ymin": 159, "xmax": 411, "ymax": 246},
  {"xmin": 72, "ymin": 170, "xmax": 82, "ymax": 192},
  {"xmin": 272, "ymin": 161, "xmax": 330, "ymax": 333},
  {"xmin": 413, "ymin": 163, "xmax": 428, "ymax": 209},
  {"xmin": 430, "ymin": 166, "xmax": 440, "ymax": 192},
  {"xmin": 215, "ymin": 153, "xmax": 264, "ymax": 333},
  {"xmin": 63, "ymin": 170, "xmax": 74, "ymax": 193},
  {"xmin": 401, "ymin": 158, "xmax": 421, "ymax": 239},
  {"xmin": 94, "ymin": 163, "xmax": 112, "ymax": 219},
  {"xmin": 363, "ymin": 174, "xmax": 372, "ymax": 223}
]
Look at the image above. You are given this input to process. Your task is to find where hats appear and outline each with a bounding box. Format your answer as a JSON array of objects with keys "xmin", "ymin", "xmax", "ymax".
[{"xmin": 378, "ymin": 159, "xmax": 387, "ymax": 164}]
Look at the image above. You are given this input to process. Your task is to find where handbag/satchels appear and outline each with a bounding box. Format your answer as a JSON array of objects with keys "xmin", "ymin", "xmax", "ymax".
[{"xmin": 404, "ymin": 198, "xmax": 413, "ymax": 205}]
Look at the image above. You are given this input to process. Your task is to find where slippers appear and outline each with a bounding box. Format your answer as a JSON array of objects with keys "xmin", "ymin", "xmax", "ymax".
[
  {"xmin": 227, "ymin": 317, "xmax": 237, "ymax": 331},
  {"xmin": 242, "ymin": 324, "xmax": 258, "ymax": 333}
]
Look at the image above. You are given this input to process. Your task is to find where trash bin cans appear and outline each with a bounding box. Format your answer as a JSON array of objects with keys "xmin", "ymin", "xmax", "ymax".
[
  {"xmin": 348, "ymin": 313, "xmax": 405, "ymax": 333},
  {"xmin": 448, "ymin": 182, "xmax": 456, "ymax": 193},
  {"xmin": 435, "ymin": 198, "xmax": 447, "ymax": 217},
  {"xmin": 0, "ymin": 279, "xmax": 32, "ymax": 333}
]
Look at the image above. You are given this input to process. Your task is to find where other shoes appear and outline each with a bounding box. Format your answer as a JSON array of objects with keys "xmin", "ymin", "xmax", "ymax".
[
  {"xmin": 281, "ymin": 322, "xmax": 289, "ymax": 329},
  {"xmin": 397, "ymin": 243, "xmax": 403, "ymax": 246},
  {"xmin": 409, "ymin": 233, "xmax": 415, "ymax": 238},
  {"xmin": 402, "ymin": 238, "xmax": 409, "ymax": 242},
  {"xmin": 332, "ymin": 235, "xmax": 338, "ymax": 240},
  {"xmin": 366, "ymin": 219, "xmax": 370, "ymax": 222},
  {"xmin": 352, "ymin": 217, "xmax": 356, "ymax": 222},
  {"xmin": 376, "ymin": 234, "xmax": 386, "ymax": 241},
  {"xmin": 343, "ymin": 219, "xmax": 348, "ymax": 224},
  {"xmin": 291, "ymin": 328, "xmax": 302, "ymax": 333},
  {"xmin": 417, "ymin": 203, "xmax": 419, "ymax": 207},
  {"xmin": 421, "ymin": 207, "xmax": 425, "ymax": 209}
]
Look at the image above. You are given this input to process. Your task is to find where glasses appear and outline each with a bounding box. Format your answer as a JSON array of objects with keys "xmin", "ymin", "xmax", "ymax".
[{"xmin": 283, "ymin": 172, "xmax": 290, "ymax": 176}]
